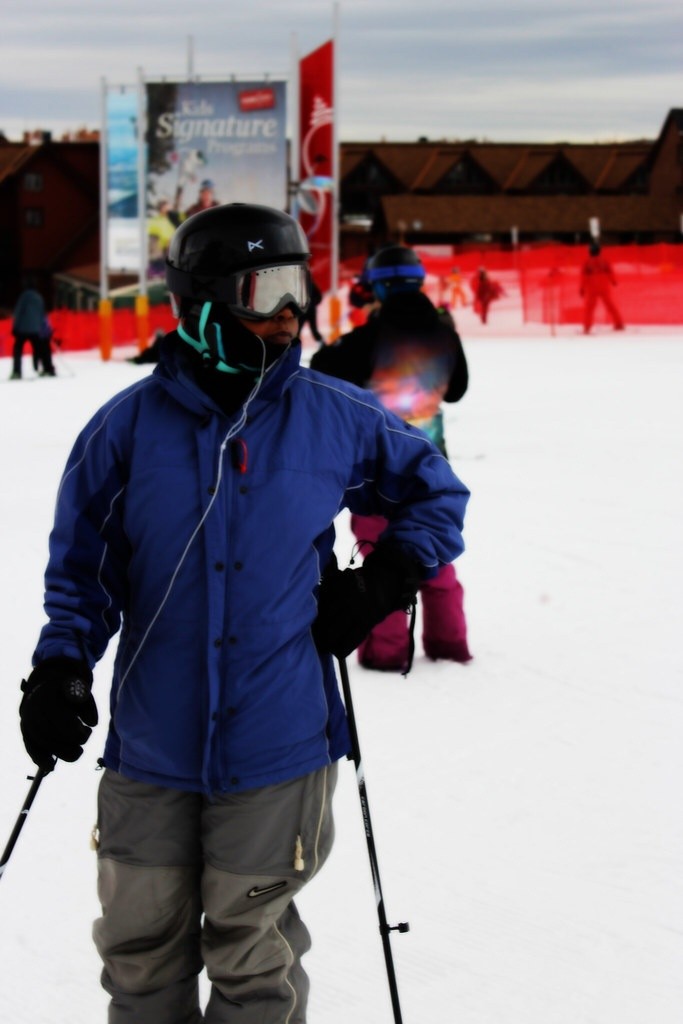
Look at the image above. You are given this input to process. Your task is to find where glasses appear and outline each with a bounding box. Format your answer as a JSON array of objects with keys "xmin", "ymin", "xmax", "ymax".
[{"xmin": 218, "ymin": 263, "xmax": 315, "ymax": 318}]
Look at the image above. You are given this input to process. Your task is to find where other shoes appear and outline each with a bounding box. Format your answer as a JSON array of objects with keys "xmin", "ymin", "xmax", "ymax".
[
  {"xmin": 11, "ymin": 373, "xmax": 23, "ymax": 379},
  {"xmin": 40, "ymin": 368, "xmax": 55, "ymax": 376}
]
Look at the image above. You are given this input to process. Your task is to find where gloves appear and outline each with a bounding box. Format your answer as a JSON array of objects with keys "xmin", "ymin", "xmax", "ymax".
[
  {"xmin": 20, "ymin": 655, "xmax": 98, "ymax": 772},
  {"xmin": 313, "ymin": 535, "xmax": 432, "ymax": 660}
]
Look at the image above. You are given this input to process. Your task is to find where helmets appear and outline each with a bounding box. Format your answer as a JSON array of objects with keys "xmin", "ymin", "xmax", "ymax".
[
  {"xmin": 367, "ymin": 245, "xmax": 425, "ymax": 300},
  {"xmin": 168, "ymin": 203, "xmax": 313, "ymax": 368}
]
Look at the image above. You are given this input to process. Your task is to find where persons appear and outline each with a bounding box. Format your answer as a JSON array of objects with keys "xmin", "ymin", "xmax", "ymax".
[
  {"xmin": 148, "ymin": 235, "xmax": 168, "ymax": 278},
  {"xmin": 18, "ymin": 198, "xmax": 470, "ymax": 1023},
  {"xmin": 447, "ymin": 266, "xmax": 470, "ymax": 310},
  {"xmin": 40, "ymin": 319, "xmax": 56, "ymax": 377},
  {"xmin": 578, "ymin": 243, "xmax": 624, "ymax": 335},
  {"xmin": 185, "ymin": 179, "xmax": 221, "ymax": 220},
  {"xmin": 475, "ymin": 267, "xmax": 491, "ymax": 324},
  {"xmin": 8, "ymin": 280, "xmax": 44, "ymax": 379},
  {"xmin": 147, "ymin": 198, "xmax": 176, "ymax": 256},
  {"xmin": 310, "ymin": 243, "xmax": 470, "ymax": 672}
]
